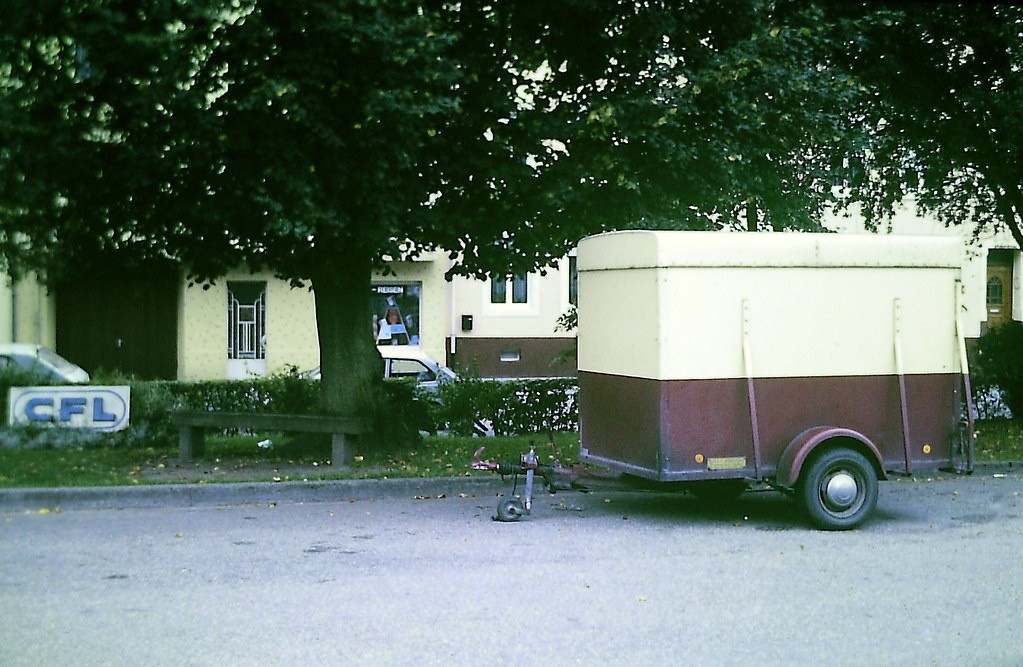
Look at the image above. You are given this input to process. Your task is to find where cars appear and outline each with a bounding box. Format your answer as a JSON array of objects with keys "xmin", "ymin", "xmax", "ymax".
[
  {"xmin": 0, "ymin": 343, "xmax": 89, "ymax": 384},
  {"xmin": 298, "ymin": 345, "xmax": 460, "ymax": 405}
]
